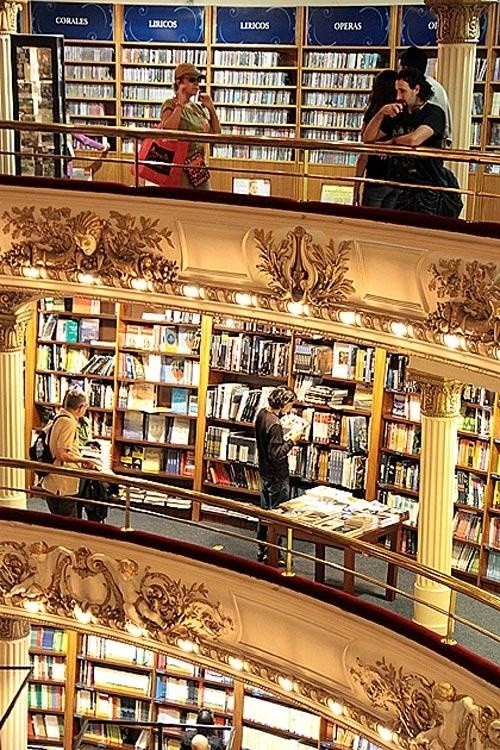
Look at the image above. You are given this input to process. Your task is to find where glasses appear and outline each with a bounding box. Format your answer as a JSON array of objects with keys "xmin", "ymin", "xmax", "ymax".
[{"xmin": 181, "ymin": 77, "xmax": 202, "ymax": 82}]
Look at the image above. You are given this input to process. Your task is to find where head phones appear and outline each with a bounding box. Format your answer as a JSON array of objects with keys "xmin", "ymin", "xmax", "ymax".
[{"xmin": 274, "ymin": 390, "xmax": 288, "ymax": 406}]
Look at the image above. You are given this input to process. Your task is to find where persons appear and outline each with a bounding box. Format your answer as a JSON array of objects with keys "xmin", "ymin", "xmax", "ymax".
[
  {"xmin": 161, "ymin": 63, "xmax": 221, "ymax": 192},
  {"xmin": 247, "ymin": 182, "xmax": 259, "ymax": 196},
  {"xmin": 353, "ymin": 70, "xmax": 401, "ymax": 210},
  {"xmin": 362, "ymin": 66, "xmax": 447, "ymax": 214}
]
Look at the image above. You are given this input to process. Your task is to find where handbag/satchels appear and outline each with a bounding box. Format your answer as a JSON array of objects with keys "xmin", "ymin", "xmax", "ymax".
[
  {"xmin": 439, "ymin": 166, "xmax": 463, "ymax": 219},
  {"xmin": 130, "ymin": 115, "xmax": 189, "ymax": 188},
  {"xmin": 183, "ymin": 152, "xmax": 210, "ymax": 186}
]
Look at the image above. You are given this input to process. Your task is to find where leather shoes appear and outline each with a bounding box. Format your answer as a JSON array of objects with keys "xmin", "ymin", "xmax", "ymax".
[{"xmin": 256, "ymin": 550, "xmax": 293, "ymax": 566}]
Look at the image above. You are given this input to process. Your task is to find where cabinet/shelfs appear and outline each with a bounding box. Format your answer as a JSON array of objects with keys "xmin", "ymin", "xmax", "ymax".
[
  {"xmin": 233, "ymin": 676, "xmax": 326, "ymax": 750},
  {"xmin": 26, "ymin": 292, "xmax": 120, "ymax": 498},
  {"xmin": 0, "ymin": 0, "xmax": 500, "ymax": 224},
  {"xmin": 110, "ymin": 298, "xmax": 293, "ymax": 522},
  {"xmin": 149, "ymin": 650, "xmax": 244, "ymax": 750},
  {"xmin": 318, "ymin": 714, "xmax": 404, "ymax": 750},
  {"xmin": 451, "ymin": 383, "xmax": 500, "ymax": 587},
  {"xmin": 26, "ymin": 619, "xmax": 158, "ymax": 750},
  {"xmin": 289, "ymin": 325, "xmax": 421, "ymax": 561}
]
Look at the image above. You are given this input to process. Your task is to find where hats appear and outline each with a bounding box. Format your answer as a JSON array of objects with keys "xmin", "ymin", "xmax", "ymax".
[{"xmin": 175, "ymin": 61, "xmax": 207, "ymax": 80}]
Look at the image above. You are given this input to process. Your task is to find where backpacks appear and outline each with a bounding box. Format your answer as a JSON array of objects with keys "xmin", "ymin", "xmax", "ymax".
[{"xmin": 28, "ymin": 415, "xmax": 71, "ymax": 475}]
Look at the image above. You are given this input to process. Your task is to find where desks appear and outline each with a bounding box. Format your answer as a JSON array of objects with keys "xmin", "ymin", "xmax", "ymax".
[{"xmin": 261, "ymin": 491, "xmax": 408, "ymax": 601}]
